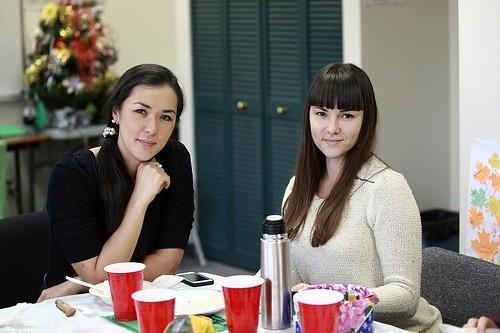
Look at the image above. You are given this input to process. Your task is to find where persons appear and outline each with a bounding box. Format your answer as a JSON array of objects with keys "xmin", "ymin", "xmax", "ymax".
[
  {"xmin": 37, "ymin": 64, "xmax": 195, "ymax": 303},
  {"xmin": 254, "ymin": 63, "xmax": 443, "ymax": 333}
]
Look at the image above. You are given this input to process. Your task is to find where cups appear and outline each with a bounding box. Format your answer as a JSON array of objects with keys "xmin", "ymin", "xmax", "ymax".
[
  {"xmin": 220, "ymin": 275, "xmax": 265, "ymax": 333},
  {"xmin": 104, "ymin": 262, "xmax": 146, "ymax": 322},
  {"xmin": 294, "ymin": 289, "xmax": 344, "ymax": 333},
  {"xmin": 131, "ymin": 289, "xmax": 177, "ymax": 333}
]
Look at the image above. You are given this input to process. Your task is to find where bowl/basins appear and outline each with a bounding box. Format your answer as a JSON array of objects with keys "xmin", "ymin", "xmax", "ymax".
[{"xmin": 89, "ymin": 279, "xmax": 157, "ymax": 305}]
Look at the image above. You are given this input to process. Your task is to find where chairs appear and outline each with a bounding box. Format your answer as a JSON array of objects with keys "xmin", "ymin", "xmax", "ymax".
[
  {"xmin": 418, "ymin": 247, "xmax": 500, "ymax": 328},
  {"xmin": 1, "ymin": 212, "xmax": 50, "ymax": 310}
]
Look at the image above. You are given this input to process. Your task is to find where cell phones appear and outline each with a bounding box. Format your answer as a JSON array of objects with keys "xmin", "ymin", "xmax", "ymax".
[{"xmin": 175, "ymin": 271, "xmax": 214, "ymax": 287}]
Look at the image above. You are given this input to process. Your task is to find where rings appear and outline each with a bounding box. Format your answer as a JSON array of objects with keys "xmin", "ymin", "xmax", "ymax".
[{"xmin": 154, "ymin": 165, "xmax": 160, "ymax": 169}]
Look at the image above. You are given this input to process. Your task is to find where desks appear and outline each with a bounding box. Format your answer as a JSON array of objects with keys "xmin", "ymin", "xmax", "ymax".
[
  {"xmin": 24, "ymin": 124, "xmax": 107, "ymax": 214},
  {"xmin": 0, "ymin": 135, "xmax": 49, "ymax": 218},
  {"xmin": 0, "ymin": 272, "xmax": 414, "ymax": 333}
]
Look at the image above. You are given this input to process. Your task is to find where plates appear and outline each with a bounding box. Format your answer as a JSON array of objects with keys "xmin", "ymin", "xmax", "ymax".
[{"xmin": 171, "ymin": 289, "xmax": 226, "ymax": 317}]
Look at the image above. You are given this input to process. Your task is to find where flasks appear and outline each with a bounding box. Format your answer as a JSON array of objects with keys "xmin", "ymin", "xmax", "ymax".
[{"xmin": 260, "ymin": 215, "xmax": 293, "ymax": 330}]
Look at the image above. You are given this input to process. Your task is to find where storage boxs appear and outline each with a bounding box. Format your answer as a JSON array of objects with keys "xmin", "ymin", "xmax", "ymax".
[{"xmin": 419, "ymin": 207, "xmax": 460, "ymax": 247}]
